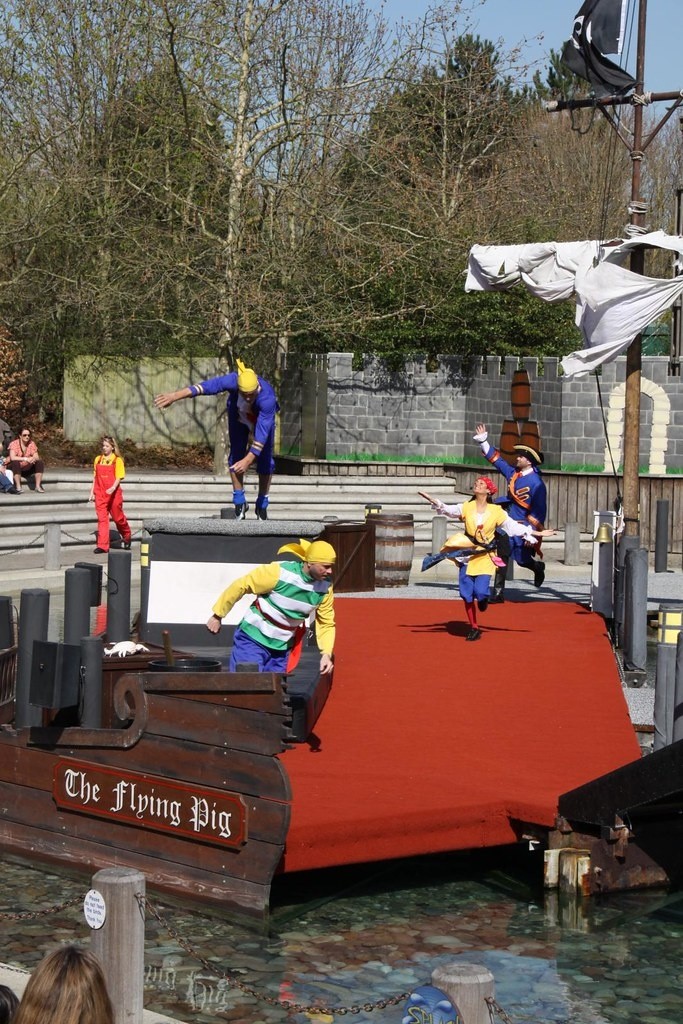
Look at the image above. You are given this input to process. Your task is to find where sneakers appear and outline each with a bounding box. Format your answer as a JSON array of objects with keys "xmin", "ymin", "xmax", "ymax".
[
  {"xmin": 255, "ymin": 507, "xmax": 267, "ymax": 520},
  {"xmin": 235, "ymin": 502, "xmax": 249, "ymax": 519}
]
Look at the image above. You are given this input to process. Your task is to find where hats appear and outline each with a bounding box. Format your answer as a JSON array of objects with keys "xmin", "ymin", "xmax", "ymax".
[{"xmin": 512, "ymin": 444, "xmax": 545, "ymax": 465}]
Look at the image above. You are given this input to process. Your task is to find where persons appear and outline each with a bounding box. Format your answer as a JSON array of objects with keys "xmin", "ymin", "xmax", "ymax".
[
  {"xmin": 88, "ymin": 435, "xmax": 132, "ymax": 554},
  {"xmin": 418, "ymin": 477, "xmax": 555, "ymax": 641},
  {"xmin": 0, "ymin": 427, "xmax": 44, "ymax": 494},
  {"xmin": 207, "ymin": 538, "xmax": 338, "ymax": 675},
  {"xmin": 0, "ymin": 943, "xmax": 114, "ymax": 1024},
  {"xmin": 154, "ymin": 358, "xmax": 282, "ymax": 521},
  {"xmin": 472, "ymin": 425, "xmax": 548, "ymax": 603}
]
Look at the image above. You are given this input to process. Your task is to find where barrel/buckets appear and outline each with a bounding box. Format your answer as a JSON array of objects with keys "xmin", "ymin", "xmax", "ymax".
[
  {"xmin": 148, "ymin": 659, "xmax": 221, "ymax": 672},
  {"xmin": 364, "ymin": 513, "xmax": 415, "ymax": 588}
]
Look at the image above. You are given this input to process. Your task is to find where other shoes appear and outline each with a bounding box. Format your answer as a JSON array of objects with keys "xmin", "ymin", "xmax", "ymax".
[
  {"xmin": 478, "ymin": 599, "xmax": 487, "ymax": 611},
  {"xmin": 93, "ymin": 548, "xmax": 109, "ymax": 554},
  {"xmin": 15, "ymin": 488, "xmax": 24, "ymax": 493},
  {"xmin": 467, "ymin": 628, "xmax": 481, "ymax": 641},
  {"xmin": 35, "ymin": 488, "xmax": 44, "ymax": 493},
  {"xmin": 124, "ymin": 540, "xmax": 131, "ymax": 549},
  {"xmin": 8, "ymin": 487, "xmax": 20, "ymax": 494}
]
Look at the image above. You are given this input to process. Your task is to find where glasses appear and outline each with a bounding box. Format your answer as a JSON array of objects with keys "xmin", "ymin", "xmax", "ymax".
[{"xmin": 23, "ymin": 434, "xmax": 30, "ymax": 437}]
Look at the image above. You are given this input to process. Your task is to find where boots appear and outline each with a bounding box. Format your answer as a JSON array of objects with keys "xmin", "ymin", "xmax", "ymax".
[
  {"xmin": 518, "ymin": 558, "xmax": 545, "ymax": 587},
  {"xmin": 488, "ymin": 568, "xmax": 505, "ymax": 603}
]
